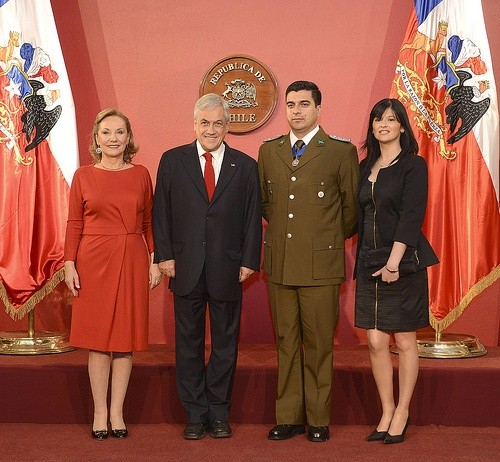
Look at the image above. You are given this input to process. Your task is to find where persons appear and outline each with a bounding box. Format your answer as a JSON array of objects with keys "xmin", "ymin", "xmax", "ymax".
[
  {"xmin": 358, "ymin": 98, "xmax": 441, "ymax": 445},
  {"xmin": 63, "ymin": 109, "xmax": 163, "ymax": 439},
  {"xmin": 153, "ymin": 94, "xmax": 262, "ymax": 440},
  {"xmin": 257, "ymin": 80, "xmax": 360, "ymax": 443}
]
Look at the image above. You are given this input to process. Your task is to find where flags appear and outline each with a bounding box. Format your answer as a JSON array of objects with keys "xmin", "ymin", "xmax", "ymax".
[
  {"xmin": 389, "ymin": 0, "xmax": 500, "ymax": 335},
  {"xmin": 1, "ymin": 0, "xmax": 81, "ymax": 321}
]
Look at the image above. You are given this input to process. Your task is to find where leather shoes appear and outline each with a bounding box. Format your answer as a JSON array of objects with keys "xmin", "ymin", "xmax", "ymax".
[
  {"xmin": 183, "ymin": 423, "xmax": 206, "ymax": 439},
  {"xmin": 207, "ymin": 418, "xmax": 232, "ymax": 438},
  {"xmin": 268, "ymin": 424, "xmax": 306, "ymax": 439},
  {"xmin": 306, "ymin": 424, "xmax": 329, "ymax": 442}
]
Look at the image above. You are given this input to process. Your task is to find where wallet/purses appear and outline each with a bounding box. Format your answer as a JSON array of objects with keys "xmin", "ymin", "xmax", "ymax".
[{"xmin": 364, "ymin": 246, "xmax": 418, "ymax": 281}]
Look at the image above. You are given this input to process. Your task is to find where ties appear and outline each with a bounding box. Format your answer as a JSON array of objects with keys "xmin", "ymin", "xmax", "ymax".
[
  {"xmin": 202, "ymin": 153, "xmax": 215, "ymax": 203},
  {"xmin": 293, "ymin": 140, "xmax": 305, "ymax": 160}
]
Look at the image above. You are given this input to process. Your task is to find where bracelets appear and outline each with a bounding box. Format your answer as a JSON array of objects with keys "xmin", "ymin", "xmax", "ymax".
[{"xmin": 385, "ymin": 267, "xmax": 400, "ymax": 274}]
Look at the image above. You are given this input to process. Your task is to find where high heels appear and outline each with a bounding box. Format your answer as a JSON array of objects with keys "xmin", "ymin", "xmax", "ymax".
[
  {"xmin": 92, "ymin": 419, "xmax": 109, "ymax": 440},
  {"xmin": 366, "ymin": 411, "xmax": 394, "ymax": 441},
  {"xmin": 383, "ymin": 411, "xmax": 410, "ymax": 443},
  {"xmin": 109, "ymin": 419, "xmax": 128, "ymax": 438}
]
